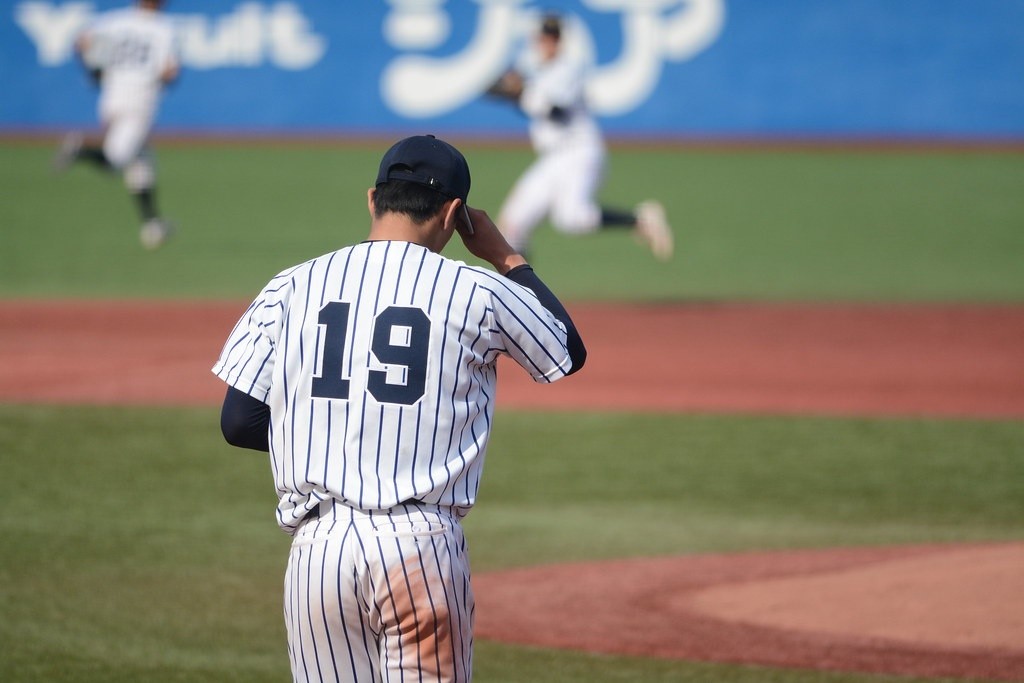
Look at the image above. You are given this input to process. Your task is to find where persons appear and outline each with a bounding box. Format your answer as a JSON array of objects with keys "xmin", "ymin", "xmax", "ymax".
[
  {"xmin": 61, "ymin": 0, "xmax": 184, "ymax": 254},
  {"xmin": 482, "ymin": 12, "xmax": 675, "ymax": 262},
  {"xmin": 220, "ymin": 134, "xmax": 588, "ymax": 683}
]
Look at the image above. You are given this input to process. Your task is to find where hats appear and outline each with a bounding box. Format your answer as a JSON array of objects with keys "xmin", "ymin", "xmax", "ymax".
[{"xmin": 376, "ymin": 134, "xmax": 474, "ymax": 236}]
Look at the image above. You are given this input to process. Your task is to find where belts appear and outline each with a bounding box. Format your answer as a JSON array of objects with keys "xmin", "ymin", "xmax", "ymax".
[{"xmin": 302, "ymin": 497, "xmax": 424, "ymax": 520}]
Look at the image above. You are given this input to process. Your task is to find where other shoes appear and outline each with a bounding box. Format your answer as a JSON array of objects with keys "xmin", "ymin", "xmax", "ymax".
[{"xmin": 640, "ymin": 200, "xmax": 674, "ymax": 258}]
